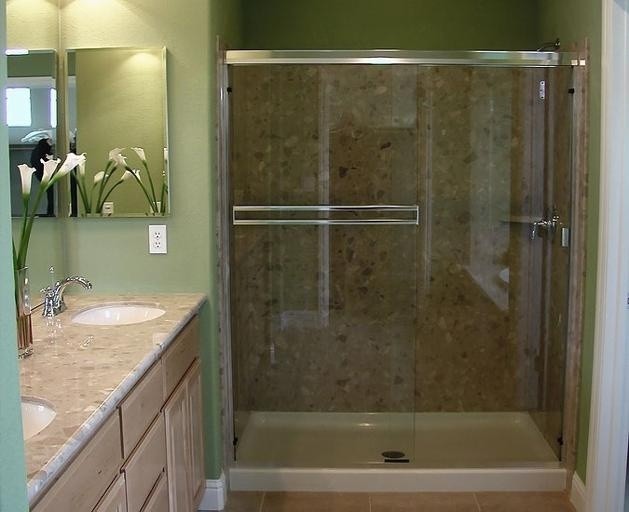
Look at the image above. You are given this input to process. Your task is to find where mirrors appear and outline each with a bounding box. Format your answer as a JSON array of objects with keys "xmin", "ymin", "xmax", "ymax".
[
  {"xmin": 7, "ymin": 48, "xmax": 58, "ymax": 220},
  {"xmin": 65, "ymin": 51, "xmax": 168, "ymax": 217}
]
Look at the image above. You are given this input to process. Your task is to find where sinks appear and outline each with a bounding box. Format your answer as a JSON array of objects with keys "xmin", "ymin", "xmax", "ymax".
[
  {"xmin": 69, "ymin": 300, "xmax": 167, "ymax": 326},
  {"xmin": 20, "ymin": 395, "xmax": 58, "ymax": 441}
]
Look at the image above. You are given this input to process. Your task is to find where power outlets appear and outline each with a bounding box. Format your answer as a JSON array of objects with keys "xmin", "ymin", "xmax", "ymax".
[{"xmin": 148, "ymin": 224, "xmax": 168, "ymax": 254}]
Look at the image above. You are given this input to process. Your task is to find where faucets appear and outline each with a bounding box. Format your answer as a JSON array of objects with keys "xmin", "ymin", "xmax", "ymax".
[
  {"xmin": 45, "ymin": 318, "xmax": 94, "ymax": 352},
  {"xmin": 39, "ymin": 265, "xmax": 93, "ymax": 317}
]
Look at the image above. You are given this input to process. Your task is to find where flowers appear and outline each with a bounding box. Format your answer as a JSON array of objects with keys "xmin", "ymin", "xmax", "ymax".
[
  {"xmin": 13, "ymin": 149, "xmax": 84, "ymax": 265},
  {"xmin": 74, "ymin": 138, "xmax": 166, "ymax": 215}
]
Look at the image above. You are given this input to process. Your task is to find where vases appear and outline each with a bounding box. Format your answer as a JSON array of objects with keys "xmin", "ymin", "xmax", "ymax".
[{"xmin": 16, "ymin": 271, "xmax": 37, "ymax": 355}]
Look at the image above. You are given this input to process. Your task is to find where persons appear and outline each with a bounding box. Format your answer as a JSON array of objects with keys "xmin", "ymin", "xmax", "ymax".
[{"xmin": 30, "ymin": 136, "xmax": 77, "ymax": 217}]
